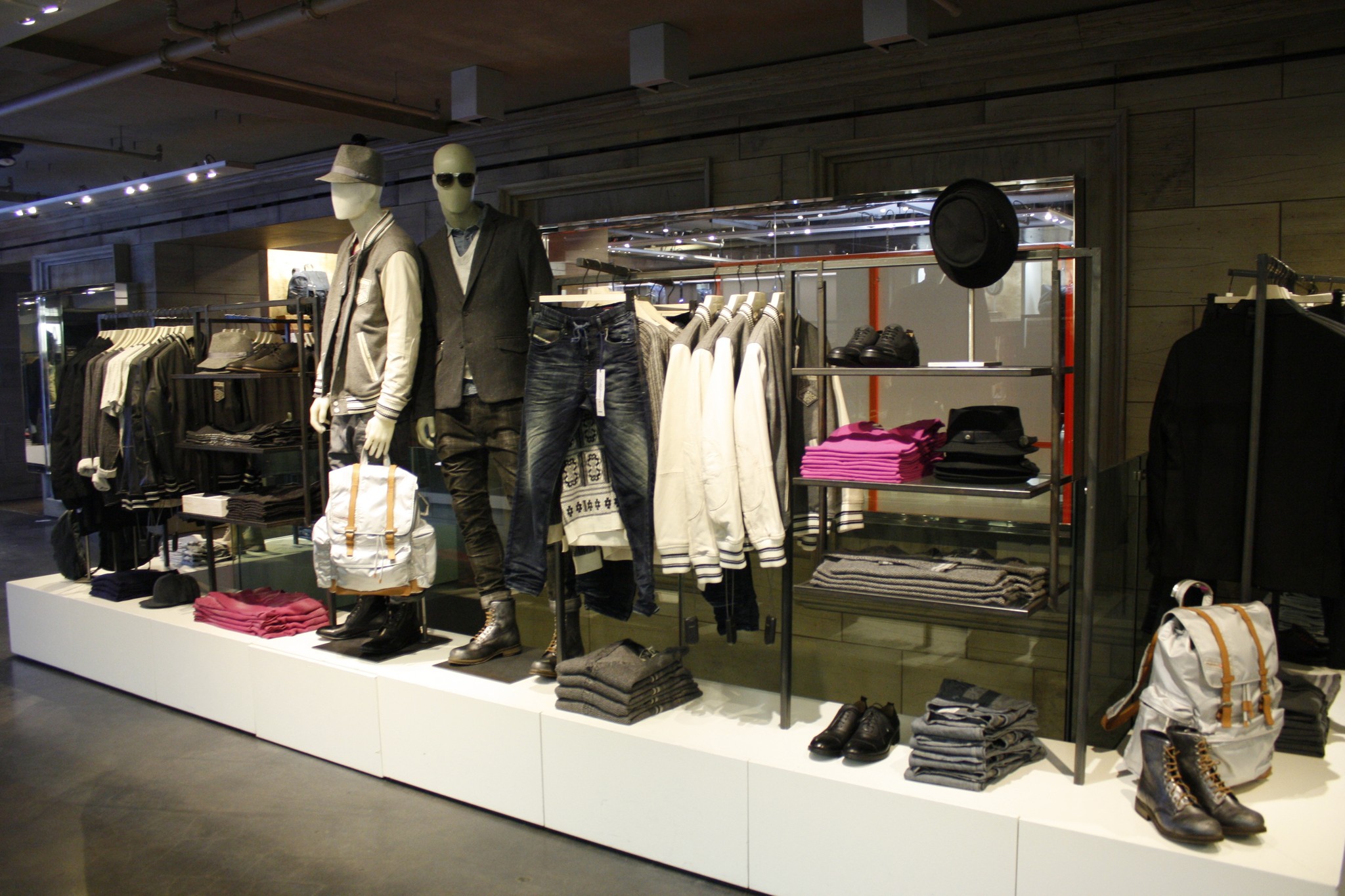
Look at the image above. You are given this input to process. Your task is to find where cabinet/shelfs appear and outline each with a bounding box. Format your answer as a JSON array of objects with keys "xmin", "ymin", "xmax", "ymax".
[
  {"xmin": 779, "ymin": 247, "xmax": 1104, "ymax": 784},
  {"xmin": 97, "ymin": 297, "xmax": 320, "ymax": 590}
]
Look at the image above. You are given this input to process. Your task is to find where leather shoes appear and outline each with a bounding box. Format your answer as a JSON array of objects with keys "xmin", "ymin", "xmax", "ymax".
[
  {"xmin": 225, "ymin": 342, "xmax": 280, "ymax": 372},
  {"xmin": 843, "ymin": 702, "xmax": 900, "ymax": 762},
  {"xmin": 825, "ymin": 326, "xmax": 882, "ymax": 367},
  {"xmin": 242, "ymin": 342, "xmax": 306, "ymax": 372},
  {"xmin": 860, "ymin": 325, "xmax": 919, "ymax": 366},
  {"xmin": 808, "ymin": 696, "xmax": 868, "ymax": 758}
]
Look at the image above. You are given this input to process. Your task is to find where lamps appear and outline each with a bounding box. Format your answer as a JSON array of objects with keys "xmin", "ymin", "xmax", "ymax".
[
  {"xmin": 79, "ymin": 185, "xmax": 92, "ymax": 203},
  {"xmin": 122, "ymin": 176, "xmax": 135, "ymax": 194},
  {"xmin": 187, "ymin": 162, "xmax": 199, "ymax": 180},
  {"xmin": 625, "ymin": 201, "xmax": 1065, "ymax": 249},
  {"xmin": 68, "ymin": 190, "xmax": 79, "ymax": 205},
  {"xmin": 204, "ymin": 154, "xmax": 217, "ymax": 178},
  {"xmin": 138, "ymin": 171, "xmax": 149, "ymax": 191}
]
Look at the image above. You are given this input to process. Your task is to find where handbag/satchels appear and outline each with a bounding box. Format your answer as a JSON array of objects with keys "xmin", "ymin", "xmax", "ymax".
[
  {"xmin": 287, "ymin": 263, "xmax": 329, "ymax": 316},
  {"xmin": 1274, "ymin": 669, "xmax": 1331, "ymax": 759}
]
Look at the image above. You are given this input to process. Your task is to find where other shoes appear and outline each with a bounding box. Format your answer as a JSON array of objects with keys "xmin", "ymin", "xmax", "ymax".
[{"xmin": 215, "ymin": 523, "xmax": 266, "ymax": 551}]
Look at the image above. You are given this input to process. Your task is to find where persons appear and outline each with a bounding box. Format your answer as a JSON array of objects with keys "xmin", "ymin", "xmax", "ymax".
[
  {"xmin": 309, "ymin": 147, "xmax": 423, "ymax": 659},
  {"xmin": 412, "ymin": 144, "xmax": 587, "ymax": 677}
]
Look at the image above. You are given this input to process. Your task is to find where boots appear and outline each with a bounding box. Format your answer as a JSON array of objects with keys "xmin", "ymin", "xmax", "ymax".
[
  {"xmin": 529, "ymin": 609, "xmax": 584, "ymax": 680},
  {"xmin": 360, "ymin": 601, "xmax": 421, "ymax": 655},
  {"xmin": 448, "ymin": 598, "xmax": 520, "ymax": 665},
  {"xmin": 1135, "ymin": 728, "xmax": 1223, "ymax": 844},
  {"xmin": 1165, "ymin": 726, "xmax": 1266, "ymax": 838},
  {"xmin": 316, "ymin": 595, "xmax": 382, "ymax": 640}
]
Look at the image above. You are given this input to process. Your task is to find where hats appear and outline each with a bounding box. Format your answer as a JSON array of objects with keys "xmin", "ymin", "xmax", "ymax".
[
  {"xmin": 929, "ymin": 179, "xmax": 1020, "ymax": 289},
  {"xmin": 197, "ymin": 331, "xmax": 255, "ymax": 369},
  {"xmin": 932, "ymin": 406, "xmax": 1039, "ymax": 485},
  {"xmin": 139, "ymin": 572, "xmax": 200, "ymax": 609},
  {"xmin": 315, "ymin": 145, "xmax": 385, "ymax": 186}
]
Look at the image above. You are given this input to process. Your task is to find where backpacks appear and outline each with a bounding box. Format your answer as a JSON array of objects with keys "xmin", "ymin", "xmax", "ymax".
[
  {"xmin": 312, "ymin": 441, "xmax": 437, "ymax": 591},
  {"xmin": 1101, "ymin": 579, "xmax": 1285, "ymax": 789}
]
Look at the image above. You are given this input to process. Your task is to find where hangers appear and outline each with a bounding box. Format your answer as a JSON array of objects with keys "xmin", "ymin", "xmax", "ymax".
[
  {"xmin": 96, "ymin": 304, "xmax": 194, "ymax": 352},
  {"xmin": 1213, "ymin": 257, "xmax": 1345, "ymax": 307},
  {"xmin": 224, "ymin": 312, "xmax": 315, "ymax": 348},
  {"xmin": 727, "ymin": 263, "xmax": 748, "ymax": 314},
  {"xmin": 747, "ymin": 262, "xmax": 768, "ymax": 317},
  {"xmin": 703, "ymin": 266, "xmax": 725, "ymax": 315},
  {"xmin": 771, "ymin": 261, "xmax": 786, "ymax": 317},
  {"xmin": 536, "ymin": 258, "xmax": 677, "ymax": 332}
]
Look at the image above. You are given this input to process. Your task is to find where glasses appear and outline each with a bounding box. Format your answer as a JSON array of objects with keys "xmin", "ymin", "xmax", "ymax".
[{"xmin": 434, "ymin": 171, "xmax": 477, "ymax": 187}]
